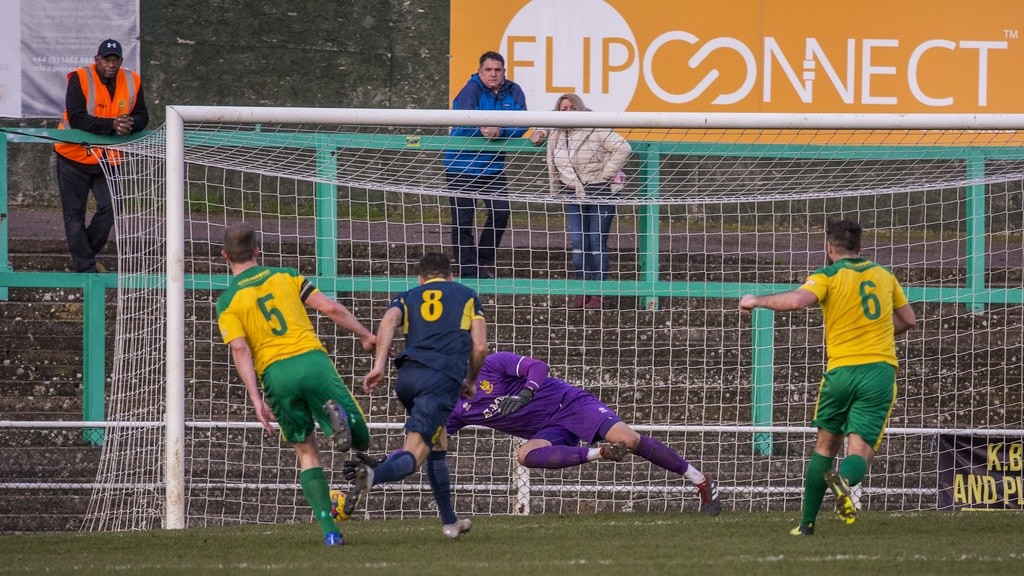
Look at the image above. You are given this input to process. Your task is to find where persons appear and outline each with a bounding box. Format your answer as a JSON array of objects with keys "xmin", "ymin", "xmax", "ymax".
[
  {"xmin": 443, "ymin": 51, "xmax": 529, "ymax": 279},
  {"xmin": 530, "ymin": 94, "xmax": 632, "ymax": 309},
  {"xmin": 343, "ymin": 250, "xmax": 488, "ymax": 541},
  {"xmin": 54, "ymin": 39, "xmax": 149, "ymax": 274},
  {"xmin": 740, "ymin": 220, "xmax": 916, "ymax": 535},
  {"xmin": 215, "ymin": 223, "xmax": 376, "ymax": 545},
  {"xmin": 445, "ymin": 352, "xmax": 721, "ymax": 516}
]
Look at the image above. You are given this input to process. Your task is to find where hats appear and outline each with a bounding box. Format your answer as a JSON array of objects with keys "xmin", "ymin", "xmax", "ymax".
[{"xmin": 99, "ymin": 38, "xmax": 122, "ymax": 59}]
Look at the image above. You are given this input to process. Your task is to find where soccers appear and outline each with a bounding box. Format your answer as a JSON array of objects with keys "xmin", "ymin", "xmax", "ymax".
[{"xmin": 327, "ymin": 490, "xmax": 352, "ymax": 523}]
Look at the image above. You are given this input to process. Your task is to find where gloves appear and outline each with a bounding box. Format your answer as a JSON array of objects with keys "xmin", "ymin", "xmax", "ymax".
[
  {"xmin": 345, "ymin": 452, "xmax": 386, "ymax": 483},
  {"xmin": 496, "ymin": 385, "xmax": 534, "ymax": 416}
]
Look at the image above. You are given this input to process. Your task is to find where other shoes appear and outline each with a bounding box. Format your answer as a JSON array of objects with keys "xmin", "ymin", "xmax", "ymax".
[{"xmin": 567, "ymin": 295, "xmax": 606, "ymax": 309}]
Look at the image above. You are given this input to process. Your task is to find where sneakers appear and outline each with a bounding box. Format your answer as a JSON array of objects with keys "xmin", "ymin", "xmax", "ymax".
[
  {"xmin": 696, "ymin": 472, "xmax": 721, "ymax": 517},
  {"xmin": 600, "ymin": 441, "xmax": 627, "ymax": 461},
  {"xmin": 789, "ymin": 521, "xmax": 814, "ymax": 535},
  {"xmin": 441, "ymin": 518, "xmax": 472, "ymax": 539},
  {"xmin": 326, "ymin": 530, "xmax": 344, "ymax": 547},
  {"xmin": 341, "ymin": 464, "xmax": 373, "ymax": 515},
  {"xmin": 823, "ymin": 470, "xmax": 856, "ymax": 524},
  {"xmin": 322, "ymin": 398, "xmax": 353, "ymax": 452}
]
